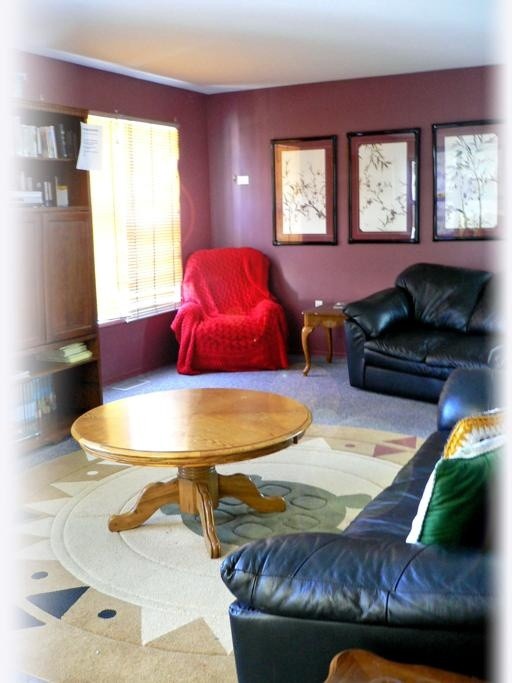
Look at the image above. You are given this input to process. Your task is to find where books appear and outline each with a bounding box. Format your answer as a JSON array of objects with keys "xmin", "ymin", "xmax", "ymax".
[
  {"xmin": 5, "ymin": 341, "xmax": 96, "ymax": 445},
  {"xmin": 9, "ymin": 108, "xmax": 79, "ymax": 206}
]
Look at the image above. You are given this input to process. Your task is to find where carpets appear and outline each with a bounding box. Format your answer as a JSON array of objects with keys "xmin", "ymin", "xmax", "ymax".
[{"xmin": 12, "ymin": 423, "xmax": 426, "ymax": 681}]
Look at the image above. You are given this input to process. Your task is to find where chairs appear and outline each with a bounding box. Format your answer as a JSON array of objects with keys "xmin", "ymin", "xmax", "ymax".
[{"xmin": 173, "ymin": 246, "xmax": 286, "ymax": 376}]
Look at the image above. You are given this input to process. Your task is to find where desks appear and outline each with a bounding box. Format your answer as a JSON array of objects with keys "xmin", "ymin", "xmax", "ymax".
[{"xmin": 301, "ymin": 299, "xmax": 358, "ymax": 375}]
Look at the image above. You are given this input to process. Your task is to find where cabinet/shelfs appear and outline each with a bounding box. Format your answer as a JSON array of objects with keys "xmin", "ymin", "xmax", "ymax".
[{"xmin": 12, "ymin": 98, "xmax": 105, "ymax": 462}]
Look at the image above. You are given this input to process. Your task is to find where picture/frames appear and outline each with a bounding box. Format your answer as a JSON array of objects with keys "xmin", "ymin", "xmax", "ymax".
[{"xmin": 269, "ymin": 119, "xmax": 502, "ymax": 247}]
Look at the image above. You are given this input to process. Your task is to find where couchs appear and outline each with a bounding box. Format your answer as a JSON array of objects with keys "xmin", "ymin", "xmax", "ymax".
[
  {"xmin": 222, "ymin": 367, "xmax": 491, "ymax": 679},
  {"xmin": 341, "ymin": 264, "xmax": 492, "ymax": 406}
]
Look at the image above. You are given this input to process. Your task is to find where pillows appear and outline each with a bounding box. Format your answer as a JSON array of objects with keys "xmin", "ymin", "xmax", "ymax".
[
  {"xmin": 404, "ymin": 434, "xmax": 503, "ymax": 553},
  {"xmin": 441, "ymin": 404, "xmax": 511, "ymax": 458}
]
